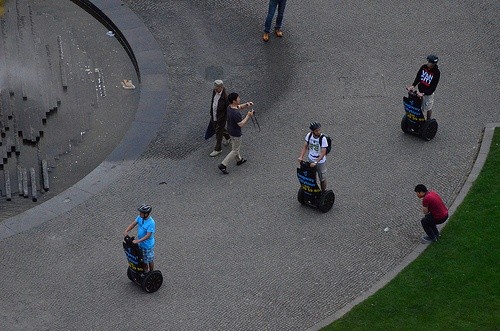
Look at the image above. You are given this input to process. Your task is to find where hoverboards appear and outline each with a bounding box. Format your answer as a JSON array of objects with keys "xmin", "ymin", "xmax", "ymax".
[
  {"xmin": 121, "ymin": 235, "xmax": 164, "ymax": 293},
  {"xmin": 295, "ymin": 159, "xmax": 336, "ymax": 213},
  {"xmin": 401, "ymin": 87, "xmax": 438, "ymax": 141}
]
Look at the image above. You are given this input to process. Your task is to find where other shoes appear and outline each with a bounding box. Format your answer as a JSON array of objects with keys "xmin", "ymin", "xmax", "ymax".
[
  {"xmin": 224, "ymin": 139, "xmax": 231, "ymax": 146},
  {"xmin": 276, "ymin": 29, "xmax": 284, "ymax": 37},
  {"xmin": 263, "ymin": 33, "xmax": 269, "ymax": 41},
  {"xmin": 237, "ymin": 158, "xmax": 247, "ymax": 165},
  {"xmin": 422, "ymin": 236, "xmax": 437, "ymax": 242},
  {"xmin": 218, "ymin": 164, "xmax": 230, "ymax": 174},
  {"xmin": 209, "ymin": 150, "xmax": 222, "ymax": 157}
]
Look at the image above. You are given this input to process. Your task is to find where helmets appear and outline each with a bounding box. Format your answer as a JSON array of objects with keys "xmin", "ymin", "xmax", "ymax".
[
  {"xmin": 308, "ymin": 121, "xmax": 322, "ymax": 130},
  {"xmin": 137, "ymin": 204, "xmax": 152, "ymax": 213}
]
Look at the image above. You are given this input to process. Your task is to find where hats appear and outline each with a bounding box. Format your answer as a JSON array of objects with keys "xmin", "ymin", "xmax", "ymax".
[
  {"xmin": 426, "ymin": 55, "xmax": 439, "ymax": 64},
  {"xmin": 212, "ymin": 79, "xmax": 224, "ymax": 89}
]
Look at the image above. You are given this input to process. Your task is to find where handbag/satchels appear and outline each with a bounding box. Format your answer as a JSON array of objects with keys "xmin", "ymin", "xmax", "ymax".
[{"xmin": 205, "ymin": 117, "xmax": 216, "ymax": 140}]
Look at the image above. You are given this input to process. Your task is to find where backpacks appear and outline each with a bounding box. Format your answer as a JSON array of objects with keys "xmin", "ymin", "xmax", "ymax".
[{"xmin": 307, "ymin": 132, "xmax": 332, "ymax": 155}]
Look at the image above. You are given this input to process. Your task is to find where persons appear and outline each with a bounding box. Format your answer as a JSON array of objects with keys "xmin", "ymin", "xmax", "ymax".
[
  {"xmin": 297, "ymin": 121, "xmax": 328, "ymax": 190},
  {"xmin": 218, "ymin": 92, "xmax": 254, "ymax": 174},
  {"xmin": 209, "ymin": 80, "xmax": 229, "ymax": 157},
  {"xmin": 414, "ymin": 184, "xmax": 449, "ymax": 242},
  {"xmin": 264, "ymin": 0, "xmax": 287, "ymax": 41},
  {"xmin": 407, "ymin": 54, "xmax": 441, "ymax": 119},
  {"xmin": 123, "ymin": 204, "xmax": 155, "ymax": 272}
]
